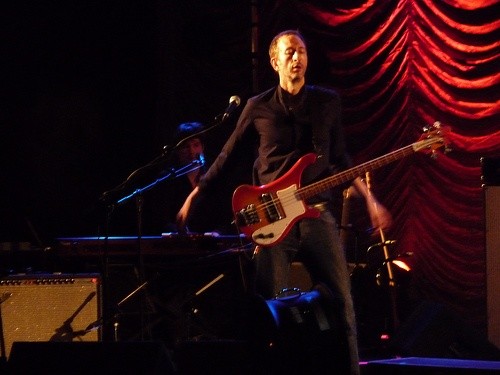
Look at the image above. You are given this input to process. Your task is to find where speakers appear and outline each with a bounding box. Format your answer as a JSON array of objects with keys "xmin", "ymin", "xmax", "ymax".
[
  {"xmin": 5, "ymin": 341, "xmax": 129, "ymax": 375},
  {"xmin": 0, "ymin": 275, "xmax": 102, "ymax": 369}
]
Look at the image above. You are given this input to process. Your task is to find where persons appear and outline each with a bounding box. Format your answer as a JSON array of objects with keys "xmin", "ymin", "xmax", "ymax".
[
  {"xmin": 179, "ymin": 29, "xmax": 392, "ymax": 375},
  {"xmin": 161, "ymin": 123, "xmax": 232, "ymax": 320}
]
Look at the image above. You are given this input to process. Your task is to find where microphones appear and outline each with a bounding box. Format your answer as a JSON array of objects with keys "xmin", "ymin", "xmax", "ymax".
[{"xmin": 223, "ymin": 96, "xmax": 241, "ymax": 119}]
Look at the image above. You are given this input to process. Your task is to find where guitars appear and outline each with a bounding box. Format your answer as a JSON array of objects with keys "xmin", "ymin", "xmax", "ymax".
[{"xmin": 230, "ymin": 120, "xmax": 453, "ymax": 249}]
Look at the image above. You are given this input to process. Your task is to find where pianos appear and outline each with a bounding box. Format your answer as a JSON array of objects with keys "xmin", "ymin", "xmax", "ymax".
[{"xmin": 55, "ymin": 232, "xmax": 259, "ymax": 342}]
